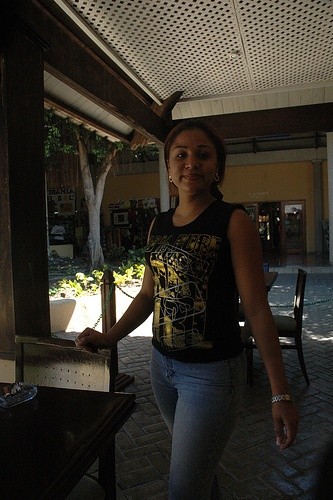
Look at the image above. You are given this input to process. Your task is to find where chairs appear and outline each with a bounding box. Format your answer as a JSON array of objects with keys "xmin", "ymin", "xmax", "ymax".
[
  {"xmin": 13, "ymin": 334, "xmax": 118, "ymax": 500},
  {"xmin": 248, "ymin": 269, "xmax": 310, "ymax": 386}
]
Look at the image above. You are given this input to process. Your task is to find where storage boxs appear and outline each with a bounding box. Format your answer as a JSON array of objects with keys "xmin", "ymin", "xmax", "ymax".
[{"xmin": 113, "ymin": 213, "xmax": 129, "ymax": 225}]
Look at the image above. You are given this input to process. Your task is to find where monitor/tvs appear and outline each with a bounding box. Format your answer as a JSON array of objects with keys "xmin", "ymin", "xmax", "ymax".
[{"xmin": 111, "ymin": 208, "xmax": 130, "ymax": 227}]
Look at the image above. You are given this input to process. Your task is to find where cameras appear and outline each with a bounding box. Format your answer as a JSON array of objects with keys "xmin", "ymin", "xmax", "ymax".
[{"xmin": 0, "ymin": 383, "xmax": 38, "ymax": 408}]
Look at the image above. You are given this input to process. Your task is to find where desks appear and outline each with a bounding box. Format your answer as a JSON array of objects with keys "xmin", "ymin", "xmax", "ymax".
[
  {"xmin": 239, "ymin": 272, "xmax": 278, "ymax": 322},
  {"xmin": 0, "ymin": 383, "xmax": 137, "ymax": 500}
]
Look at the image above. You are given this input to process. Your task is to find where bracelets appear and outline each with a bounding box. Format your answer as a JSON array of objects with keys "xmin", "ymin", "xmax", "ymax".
[{"xmin": 271, "ymin": 394, "xmax": 295, "ymax": 403}]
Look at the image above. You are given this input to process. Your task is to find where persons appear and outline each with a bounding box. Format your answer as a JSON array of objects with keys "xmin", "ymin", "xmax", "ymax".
[{"xmin": 74, "ymin": 120, "xmax": 299, "ymax": 499}]
